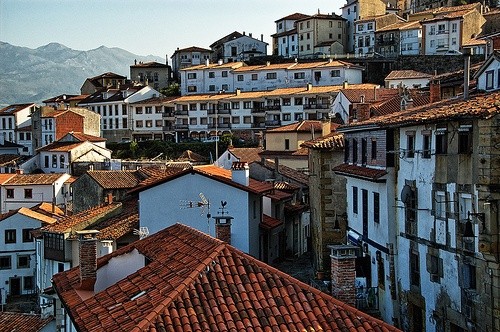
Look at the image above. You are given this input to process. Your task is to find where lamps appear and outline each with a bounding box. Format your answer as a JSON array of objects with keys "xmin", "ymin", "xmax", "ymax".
[{"xmin": 461, "ymin": 211, "xmax": 486, "ymax": 244}]
